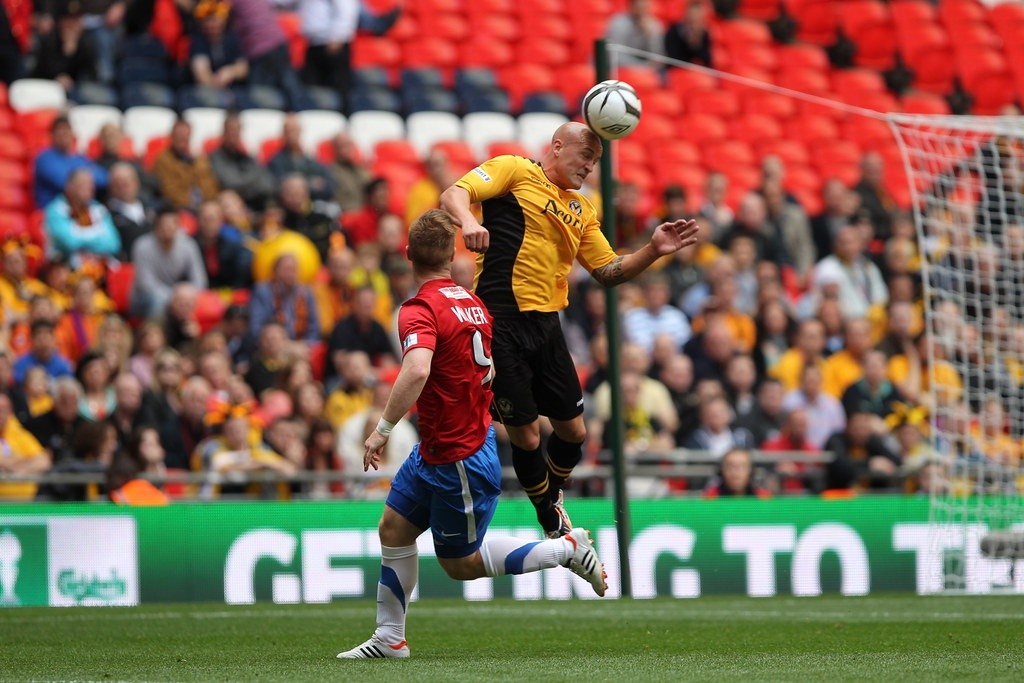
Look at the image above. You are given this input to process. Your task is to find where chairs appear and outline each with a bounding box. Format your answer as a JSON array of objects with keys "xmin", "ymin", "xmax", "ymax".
[{"xmin": 0, "ymin": 0, "xmax": 1024, "ymax": 337}]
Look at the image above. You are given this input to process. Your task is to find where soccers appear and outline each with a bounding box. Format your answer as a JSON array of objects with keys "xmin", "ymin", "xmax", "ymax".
[{"xmin": 582, "ymin": 78, "xmax": 643, "ymax": 139}]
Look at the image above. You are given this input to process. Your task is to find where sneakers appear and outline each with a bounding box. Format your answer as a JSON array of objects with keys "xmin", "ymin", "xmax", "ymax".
[
  {"xmin": 336, "ymin": 633, "xmax": 410, "ymax": 659},
  {"xmin": 562, "ymin": 528, "xmax": 608, "ymax": 596},
  {"xmin": 553, "ymin": 489, "xmax": 564, "ymax": 509},
  {"xmin": 544, "ymin": 505, "xmax": 573, "ymax": 539}
]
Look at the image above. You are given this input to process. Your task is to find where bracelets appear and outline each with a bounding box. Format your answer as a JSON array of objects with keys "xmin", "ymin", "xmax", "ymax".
[{"xmin": 376, "ymin": 417, "xmax": 395, "ymax": 437}]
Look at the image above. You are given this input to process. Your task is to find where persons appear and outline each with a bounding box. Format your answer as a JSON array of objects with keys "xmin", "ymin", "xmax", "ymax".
[
  {"xmin": 335, "ymin": 208, "xmax": 609, "ymax": 657},
  {"xmin": 604, "ymin": 0, "xmax": 711, "ymax": 81},
  {"xmin": 25, "ymin": 0, "xmax": 403, "ymax": 87},
  {"xmin": 0, "ymin": 107, "xmax": 607, "ymax": 503},
  {"xmin": 614, "ymin": 105, "xmax": 1024, "ymax": 502},
  {"xmin": 439, "ymin": 122, "xmax": 699, "ymax": 540}
]
